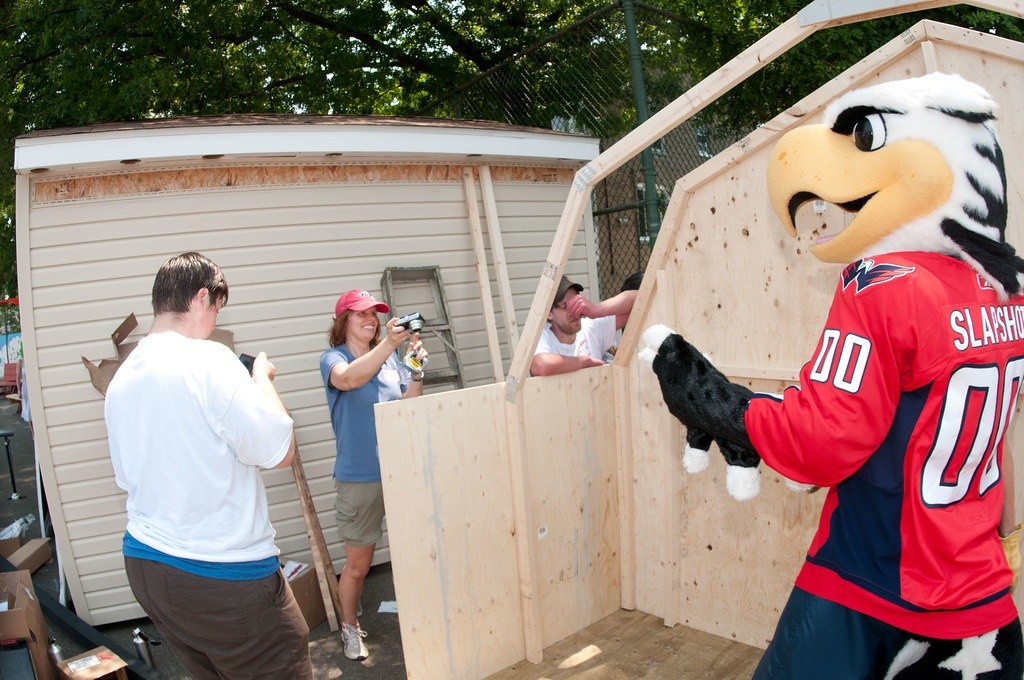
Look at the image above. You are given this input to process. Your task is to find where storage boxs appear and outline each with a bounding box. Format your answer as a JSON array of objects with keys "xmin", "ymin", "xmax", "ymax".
[
  {"xmin": 0, "ymin": 528, "xmax": 23, "ymax": 559},
  {"xmin": 55, "ymin": 645, "xmax": 128, "ymax": 680},
  {"xmin": 0, "ymin": 569, "xmax": 57, "ymax": 680},
  {"xmin": 6, "ymin": 538, "xmax": 52, "ymax": 574},
  {"xmin": 278, "ymin": 556, "xmax": 327, "ymax": 631}
]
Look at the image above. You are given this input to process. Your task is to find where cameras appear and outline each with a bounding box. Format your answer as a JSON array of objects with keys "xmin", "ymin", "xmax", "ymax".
[
  {"xmin": 238, "ymin": 353, "xmax": 256, "ymax": 376},
  {"xmin": 394, "ymin": 312, "xmax": 426, "ymax": 333}
]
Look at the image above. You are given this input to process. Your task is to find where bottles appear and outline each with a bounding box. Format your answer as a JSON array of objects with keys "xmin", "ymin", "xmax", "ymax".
[
  {"xmin": 46, "ymin": 637, "xmax": 64, "ymax": 669},
  {"xmin": 133, "ymin": 628, "xmax": 156, "ymax": 669},
  {"xmin": 0, "ymin": 513, "xmax": 35, "ymax": 539}
]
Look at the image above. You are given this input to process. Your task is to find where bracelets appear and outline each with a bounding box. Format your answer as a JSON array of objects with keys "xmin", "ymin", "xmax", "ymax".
[{"xmin": 409, "ymin": 371, "xmax": 424, "ymax": 381}]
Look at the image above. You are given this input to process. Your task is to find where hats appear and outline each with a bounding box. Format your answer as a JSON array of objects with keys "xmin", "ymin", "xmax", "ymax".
[
  {"xmin": 554, "ymin": 275, "xmax": 584, "ymax": 304},
  {"xmin": 621, "ymin": 271, "xmax": 644, "ymax": 292},
  {"xmin": 335, "ymin": 289, "xmax": 390, "ymax": 318}
]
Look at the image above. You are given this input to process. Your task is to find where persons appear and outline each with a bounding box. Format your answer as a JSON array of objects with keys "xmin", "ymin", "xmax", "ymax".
[
  {"xmin": 529, "ymin": 273, "xmax": 641, "ymax": 376},
  {"xmin": 104, "ymin": 252, "xmax": 313, "ymax": 680},
  {"xmin": 319, "ymin": 289, "xmax": 429, "ymax": 661}
]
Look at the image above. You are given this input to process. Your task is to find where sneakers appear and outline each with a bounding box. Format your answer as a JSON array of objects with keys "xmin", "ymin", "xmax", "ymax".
[{"xmin": 341, "ymin": 623, "xmax": 369, "ymax": 660}]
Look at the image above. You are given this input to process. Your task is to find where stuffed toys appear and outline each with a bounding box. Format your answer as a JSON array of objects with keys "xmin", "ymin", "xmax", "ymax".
[{"xmin": 640, "ymin": 73, "xmax": 1024, "ymax": 680}]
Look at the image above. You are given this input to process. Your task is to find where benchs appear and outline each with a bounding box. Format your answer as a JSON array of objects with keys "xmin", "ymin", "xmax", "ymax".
[{"xmin": 0, "ymin": 362, "xmax": 23, "ymax": 394}]
